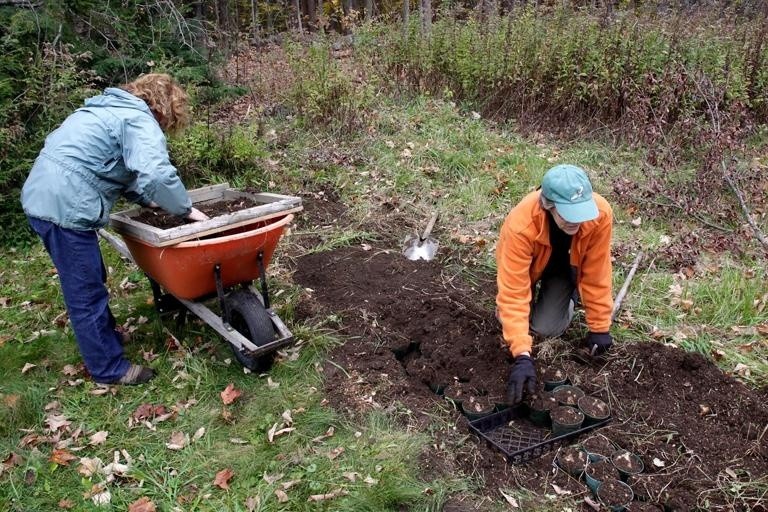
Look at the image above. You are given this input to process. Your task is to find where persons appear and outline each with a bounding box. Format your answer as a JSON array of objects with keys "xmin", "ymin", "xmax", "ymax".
[
  {"xmin": 493, "ymin": 163, "xmax": 615, "ymax": 409},
  {"xmin": 17, "ymin": 71, "xmax": 209, "ymax": 387}
]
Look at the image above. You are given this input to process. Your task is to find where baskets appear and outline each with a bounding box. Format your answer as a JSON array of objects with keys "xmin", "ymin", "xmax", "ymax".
[{"xmin": 468, "ymin": 400, "xmax": 613, "ymax": 467}]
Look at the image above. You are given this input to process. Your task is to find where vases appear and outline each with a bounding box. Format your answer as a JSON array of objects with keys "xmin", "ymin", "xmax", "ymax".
[
  {"xmin": 529, "ymin": 373, "xmax": 645, "ymax": 510},
  {"xmin": 432, "ymin": 380, "xmax": 495, "ymax": 419}
]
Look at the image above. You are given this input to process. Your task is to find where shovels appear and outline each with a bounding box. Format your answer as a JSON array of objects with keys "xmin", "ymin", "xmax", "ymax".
[{"xmin": 402, "ymin": 207, "xmax": 440, "ymax": 263}]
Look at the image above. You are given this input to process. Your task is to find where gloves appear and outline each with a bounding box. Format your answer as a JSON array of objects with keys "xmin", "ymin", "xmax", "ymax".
[
  {"xmin": 506, "ymin": 354, "xmax": 537, "ymax": 407},
  {"xmin": 585, "ymin": 331, "xmax": 613, "ymax": 356}
]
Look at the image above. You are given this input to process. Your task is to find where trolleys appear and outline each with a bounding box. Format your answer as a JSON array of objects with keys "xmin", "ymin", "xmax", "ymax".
[{"xmin": 96, "ymin": 179, "xmax": 305, "ymax": 373}]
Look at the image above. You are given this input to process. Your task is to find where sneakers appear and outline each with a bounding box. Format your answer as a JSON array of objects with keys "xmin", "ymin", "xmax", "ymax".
[
  {"xmin": 94, "ymin": 361, "xmax": 157, "ymax": 386},
  {"xmin": 117, "ymin": 330, "xmax": 145, "ymax": 347}
]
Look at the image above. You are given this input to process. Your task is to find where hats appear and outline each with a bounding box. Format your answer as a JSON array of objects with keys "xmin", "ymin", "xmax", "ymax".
[{"xmin": 542, "ymin": 164, "xmax": 600, "ymax": 225}]
